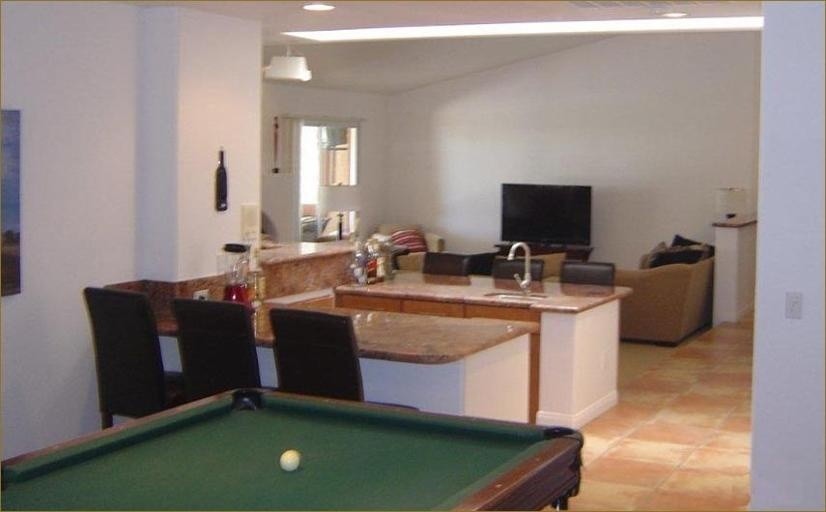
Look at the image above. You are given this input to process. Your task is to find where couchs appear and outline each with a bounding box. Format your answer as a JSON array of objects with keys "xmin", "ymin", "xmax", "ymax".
[{"xmin": 611, "ymin": 233, "xmax": 715, "ymax": 347}]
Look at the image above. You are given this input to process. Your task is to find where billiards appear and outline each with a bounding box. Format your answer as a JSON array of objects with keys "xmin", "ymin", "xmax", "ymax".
[{"xmin": 280, "ymin": 449, "xmax": 300, "ymax": 470}]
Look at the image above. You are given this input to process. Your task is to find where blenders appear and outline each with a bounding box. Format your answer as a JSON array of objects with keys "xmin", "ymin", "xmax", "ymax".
[{"xmin": 215, "ymin": 242, "xmax": 252, "ymax": 307}]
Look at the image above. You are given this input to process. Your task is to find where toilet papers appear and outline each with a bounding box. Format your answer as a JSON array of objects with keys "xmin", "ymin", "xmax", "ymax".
[{"xmin": 348, "ymin": 210, "xmax": 358, "ymax": 234}]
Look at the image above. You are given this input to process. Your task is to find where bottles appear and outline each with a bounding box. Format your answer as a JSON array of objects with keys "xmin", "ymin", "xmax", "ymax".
[{"xmin": 249, "ymin": 248, "xmax": 266, "ymax": 302}]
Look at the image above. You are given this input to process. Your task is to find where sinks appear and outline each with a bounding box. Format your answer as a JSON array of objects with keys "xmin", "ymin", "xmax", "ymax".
[{"xmin": 485, "ymin": 292, "xmax": 549, "ymax": 303}]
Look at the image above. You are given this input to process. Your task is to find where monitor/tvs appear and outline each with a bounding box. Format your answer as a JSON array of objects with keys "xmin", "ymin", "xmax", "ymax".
[{"xmin": 501, "ymin": 183, "xmax": 590, "ymax": 248}]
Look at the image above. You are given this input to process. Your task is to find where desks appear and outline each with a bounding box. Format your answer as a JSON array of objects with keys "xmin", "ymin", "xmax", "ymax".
[{"xmin": 0, "ymin": 386, "xmax": 585, "ymax": 511}]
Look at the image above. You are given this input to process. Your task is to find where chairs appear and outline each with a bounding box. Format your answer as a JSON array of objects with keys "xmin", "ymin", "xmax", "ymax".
[
  {"xmin": 265, "ymin": 302, "xmax": 410, "ymax": 409},
  {"xmin": 170, "ymin": 297, "xmax": 262, "ymax": 392},
  {"xmin": 83, "ymin": 285, "xmax": 197, "ymax": 430},
  {"xmin": 423, "ymin": 250, "xmax": 615, "ymax": 291},
  {"xmin": 379, "ymin": 222, "xmax": 445, "ymax": 273}
]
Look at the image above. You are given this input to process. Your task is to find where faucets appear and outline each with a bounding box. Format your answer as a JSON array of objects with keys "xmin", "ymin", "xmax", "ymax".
[{"xmin": 506, "ymin": 241, "xmax": 533, "ymax": 292}]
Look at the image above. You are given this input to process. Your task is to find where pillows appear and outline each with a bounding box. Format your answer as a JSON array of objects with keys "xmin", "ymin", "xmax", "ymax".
[
  {"xmin": 639, "ymin": 234, "xmax": 714, "ymax": 267},
  {"xmin": 389, "ymin": 229, "xmax": 427, "ymax": 252}
]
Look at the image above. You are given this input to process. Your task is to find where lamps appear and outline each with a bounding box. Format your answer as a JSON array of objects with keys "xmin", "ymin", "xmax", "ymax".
[{"xmin": 267, "ymin": 43, "xmax": 312, "ymax": 83}]
